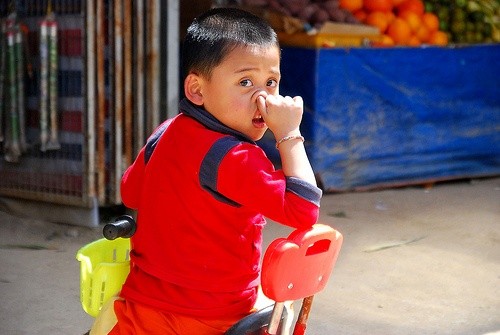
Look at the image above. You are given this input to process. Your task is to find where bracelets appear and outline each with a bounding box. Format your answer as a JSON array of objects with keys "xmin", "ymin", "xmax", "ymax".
[{"xmin": 275, "ymin": 135, "xmax": 305, "ymax": 150}]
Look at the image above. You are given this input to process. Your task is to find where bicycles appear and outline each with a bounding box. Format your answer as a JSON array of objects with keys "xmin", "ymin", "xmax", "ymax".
[{"xmin": 75, "ymin": 214, "xmax": 343, "ymax": 335}]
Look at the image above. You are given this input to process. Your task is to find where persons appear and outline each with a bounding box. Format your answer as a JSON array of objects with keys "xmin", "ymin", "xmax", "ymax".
[{"xmin": 84, "ymin": 8, "xmax": 322, "ymax": 335}]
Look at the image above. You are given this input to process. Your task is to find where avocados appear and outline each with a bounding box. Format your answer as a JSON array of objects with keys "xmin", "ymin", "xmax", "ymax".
[{"xmin": 422, "ymin": 0, "xmax": 492, "ymax": 45}]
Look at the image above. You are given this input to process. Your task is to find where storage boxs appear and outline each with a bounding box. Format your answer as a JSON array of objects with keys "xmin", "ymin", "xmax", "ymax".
[{"xmin": 277, "ymin": 21, "xmax": 379, "ymax": 47}]
